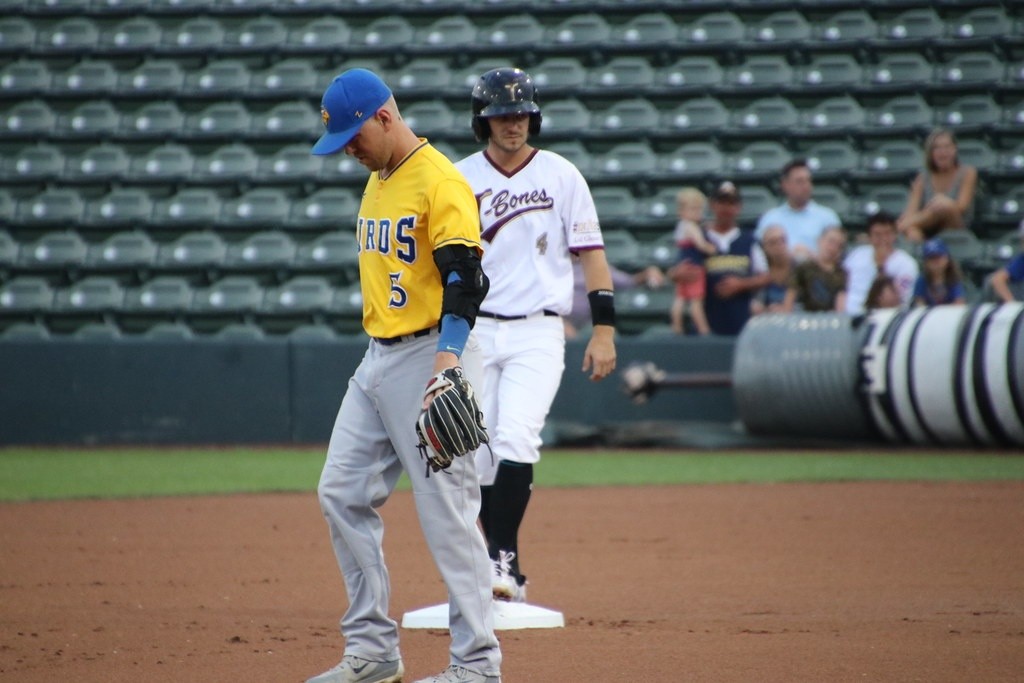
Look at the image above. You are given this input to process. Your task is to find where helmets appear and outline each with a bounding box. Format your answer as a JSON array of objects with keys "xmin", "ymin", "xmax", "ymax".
[{"xmin": 470, "ymin": 68, "xmax": 542, "ymax": 142}]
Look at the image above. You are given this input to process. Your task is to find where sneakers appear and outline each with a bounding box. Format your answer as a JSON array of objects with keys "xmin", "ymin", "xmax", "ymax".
[
  {"xmin": 491, "ymin": 550, "xmax": 527, "ymax": 603},
  {"xmin": 305, "ymin": 656, "xmax": 404, "ymax": 683},
  {"xmin": 412, "ymin": 665, "xmax": 501, "ymax": 683}
]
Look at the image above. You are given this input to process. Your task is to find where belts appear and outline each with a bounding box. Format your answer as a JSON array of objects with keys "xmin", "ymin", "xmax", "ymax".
[
  {"xmin": 373, "ymin": 326, "xmax": 441, "ymax": 345},
  {"xmin": 476, "ymin": 309, "xmax": 560, "ymax": 319}
]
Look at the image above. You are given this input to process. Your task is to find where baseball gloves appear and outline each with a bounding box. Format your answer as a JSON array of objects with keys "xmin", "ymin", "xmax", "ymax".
[{"xmin": 415, "ymin": 366, "xmax": 489, "ymax": 471}]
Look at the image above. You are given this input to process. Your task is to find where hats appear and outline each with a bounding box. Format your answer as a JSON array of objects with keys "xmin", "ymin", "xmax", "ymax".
[
  {"xmin": 311, "ymin": 68, "xmax": 392, "ymax": 156},
  {"xmin": 713, "ymin": 178, "xmax": 742, "ymax": 200},
  {"xmin": 923, "ymin": 239, "xmax": 945, "ymax": 256}
]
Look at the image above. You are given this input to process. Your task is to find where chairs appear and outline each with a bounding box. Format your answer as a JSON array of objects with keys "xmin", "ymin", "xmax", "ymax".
[{"xmin": 0, "ymin": 2, "xmax": 1024, "ymax": 337}]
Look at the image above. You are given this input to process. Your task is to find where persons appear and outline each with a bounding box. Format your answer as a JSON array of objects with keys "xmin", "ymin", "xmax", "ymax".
[
  {"xmin": 452, "ymin": 66, "xmax": 616, "ymax": 601},
  {"xmin": 562, "ymin": 125, "xmax": 1023, "ymax": 341},
  {"xmin": 304, "ymin": 66, "xmax": 502, "ymax": 683}
]
